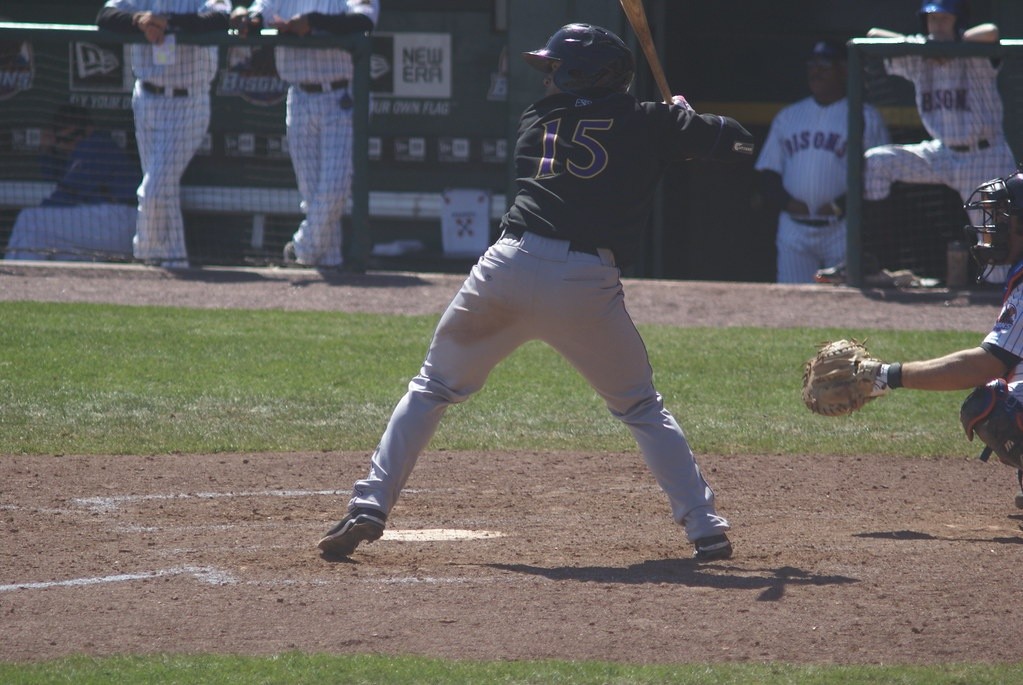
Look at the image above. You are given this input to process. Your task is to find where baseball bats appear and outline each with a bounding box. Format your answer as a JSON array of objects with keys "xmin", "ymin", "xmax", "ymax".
[{"xmin": 620, "ymin": 0, "xmax": 694, "ymax": 162}]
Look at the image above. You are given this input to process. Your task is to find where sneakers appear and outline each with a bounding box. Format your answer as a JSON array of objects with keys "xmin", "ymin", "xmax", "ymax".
[
  {"xmin": 317, "ymin": 508, "xmax": 386, "ymax": 556},
  {"xmin": 695, "ymin": 533, "xmax": 732, "ymax": 560}
]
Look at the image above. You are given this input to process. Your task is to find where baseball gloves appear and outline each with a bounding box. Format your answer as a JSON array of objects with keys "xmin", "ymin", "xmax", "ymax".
[{"xmin": 800, "ymin": 339, "xmax": 883, "ymax": 418}]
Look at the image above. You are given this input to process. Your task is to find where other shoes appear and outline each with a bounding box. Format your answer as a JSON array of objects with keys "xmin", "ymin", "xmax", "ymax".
[{"xmin": 283, "ymin": 242, "xmax": 296, "ymax": 265}]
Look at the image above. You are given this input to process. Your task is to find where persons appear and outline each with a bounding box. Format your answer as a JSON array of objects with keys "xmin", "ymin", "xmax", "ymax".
[
  {"xmin": 815, "ymin": 0, "xmax": 1019, "ymax": 289},
  {"xmin": 229, "ymin": 0, "xmax": 379, "ymax": 266},
  {"xmin": 316, "ymin": 23, "xmax": 755, "ymax": 562},
  {"xmin": 4, "ymin": 105, "xmax": 140, "ymax": 260},
  {"xmin": 813, "ymin": 172, "xmax": 1023, "ymax": 498},
  {"xmin": 747, "ymin": 42, "xmax": 891, "ymax": 282},
  {"xmin": 95, "ymin": 0, "xmax": 232, "ymax": 268}
]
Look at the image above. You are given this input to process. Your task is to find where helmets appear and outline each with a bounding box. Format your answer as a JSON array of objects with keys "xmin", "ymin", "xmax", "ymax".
[
  {"xmin": 987, "ymin": 173, "xmax": 1023, "ymax": 209},
  {"xmin": 918, "ymin": 0, "xmax": 961, "ymax": 33},
  {"xmin": 522, "ymin": 23, "xmax": 635, "ymax": 98}
]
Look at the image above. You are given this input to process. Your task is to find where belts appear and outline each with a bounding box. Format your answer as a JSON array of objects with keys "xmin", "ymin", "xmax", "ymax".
[
  {"xmin": 790, "ymin": 218, "xmax": 839, "ymax": 226},
  {"xmin": 142, "ymin": 82, "xmax": 187, "ymax": 98},
  {"xmin": 949, "ymin": 140, "xmax": 989, "ymax": 152},
  {"xmin": 301, "ymin": 79, "xmax": 347, "ymax": 93},
  {"xmin": 505, "ymin": 228, "xmax": 598, "ymax": 255}
]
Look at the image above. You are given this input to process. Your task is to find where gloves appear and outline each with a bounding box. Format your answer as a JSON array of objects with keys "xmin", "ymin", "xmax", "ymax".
[{"xmin": 866, "ymin": 361, "xmax": 904, "ymax": 397}]
